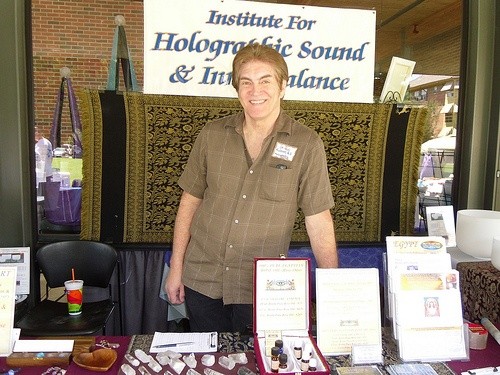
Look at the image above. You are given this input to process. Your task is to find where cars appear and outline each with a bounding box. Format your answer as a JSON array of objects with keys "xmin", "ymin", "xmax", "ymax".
[{"xmin": 53, "ymin": 148, "xmax": 69, "ymax": 157}]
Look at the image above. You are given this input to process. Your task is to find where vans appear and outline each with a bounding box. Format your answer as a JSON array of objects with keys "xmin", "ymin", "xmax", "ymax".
[{"xmin": 61, "ymin": 144, "xmax": 72, "ymax": 155}]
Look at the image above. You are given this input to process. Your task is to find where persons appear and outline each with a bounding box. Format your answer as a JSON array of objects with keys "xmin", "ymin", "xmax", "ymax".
[
  {"xmin": 165, "ymin": 42, "xmax": 338, "ymax": 332},
  {"xmin": 35, "ymin": 126, "xmax": 52, "ymax": 218}
]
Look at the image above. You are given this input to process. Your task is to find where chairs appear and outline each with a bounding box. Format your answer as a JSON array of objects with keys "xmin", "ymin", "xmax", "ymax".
[{"xmin": 15, "ymin": 241, "xmax": 119, "ymax": 336}]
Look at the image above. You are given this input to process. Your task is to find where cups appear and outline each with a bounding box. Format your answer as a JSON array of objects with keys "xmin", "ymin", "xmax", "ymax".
[{"xmin": 65, "ymin": 280, "xmax": 84, "ymax": 315}]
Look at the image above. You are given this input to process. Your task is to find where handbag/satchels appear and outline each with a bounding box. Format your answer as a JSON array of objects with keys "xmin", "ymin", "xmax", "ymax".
[{"xmin": 40, "ymin": 74, "xmax": 90, "ymax": 227}]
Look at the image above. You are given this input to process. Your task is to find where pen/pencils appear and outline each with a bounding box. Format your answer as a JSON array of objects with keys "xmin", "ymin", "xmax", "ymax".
[{"xmin": 153, "ymin": 341, "xmax": 195, "ymax": 348}]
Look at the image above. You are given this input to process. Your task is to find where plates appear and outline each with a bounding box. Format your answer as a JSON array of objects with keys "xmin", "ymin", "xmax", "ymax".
[{"xmin": 74, "ymin": 348, "xmax": 118, "ymax": 371}]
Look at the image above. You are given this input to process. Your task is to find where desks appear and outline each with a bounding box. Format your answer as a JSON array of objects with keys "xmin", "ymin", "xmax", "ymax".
[
  {"xmin": 0, "ymin": 332, "xmax": 500, "ymax": 375},
  {"xmin": 456, "ymin": 261, "xmax": 500, "ymax": 324}
]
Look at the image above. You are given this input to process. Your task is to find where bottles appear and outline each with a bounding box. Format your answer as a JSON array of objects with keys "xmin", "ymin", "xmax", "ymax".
[
  {"xmin": 294, "ymin": 340, "xmax": 303, "ymax": 360},
  {"xmin": 270, "ymin": 340, "xmax": 289, "ymax": 373},
  {"xmin": 300, "ymin": 343, "xmax": 320, "ymax": 372}
]
